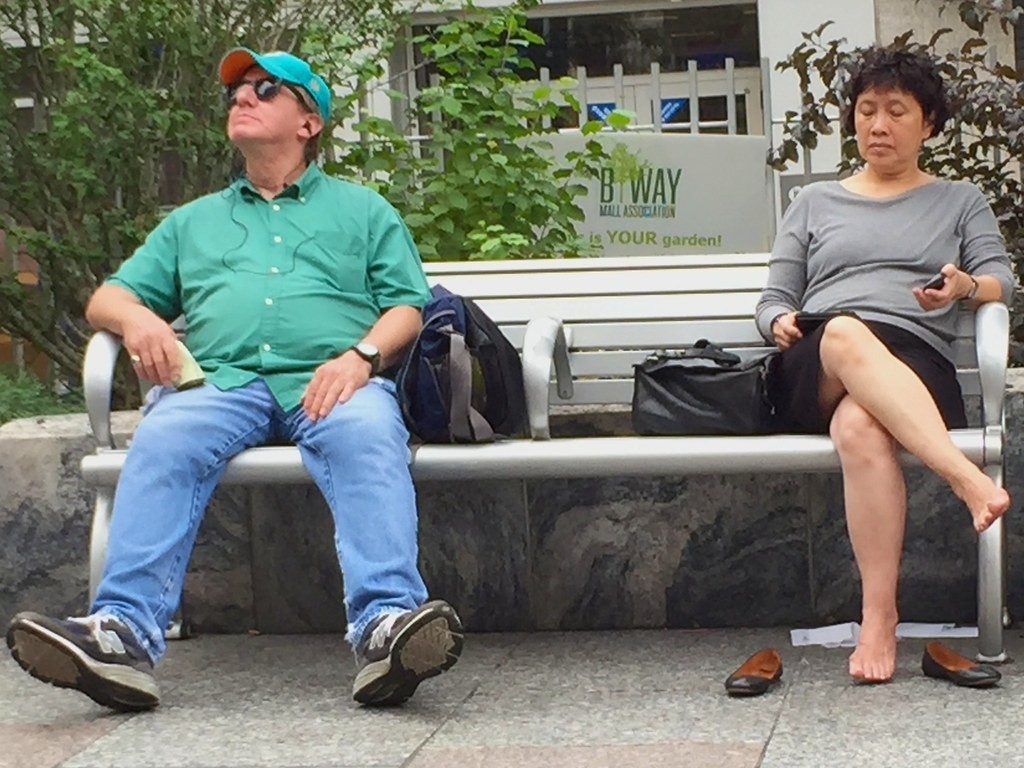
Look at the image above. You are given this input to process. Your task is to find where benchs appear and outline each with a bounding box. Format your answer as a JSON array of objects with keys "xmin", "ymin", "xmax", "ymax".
[{"xmin": 77, "ymin": 250, "xmax": 1013, "ymax": 664}]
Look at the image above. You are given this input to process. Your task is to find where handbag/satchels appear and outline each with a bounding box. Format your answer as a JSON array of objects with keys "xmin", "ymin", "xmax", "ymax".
[
  {"xmin": 394, "ymin": 284, "xmax": 526, "ymax": 446},
  {"xmin": 628, "ymin": 338, "xmax": 778, "ymax": 439}
]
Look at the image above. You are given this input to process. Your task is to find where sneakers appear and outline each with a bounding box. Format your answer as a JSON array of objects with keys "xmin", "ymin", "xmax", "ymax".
[
  {"xmin": 5, "ymin": 608, "xmax": 159, "ymax": 714},
  {"xmin": 348, "ymin": 599, "xmax": 465, "ymax": 708}
]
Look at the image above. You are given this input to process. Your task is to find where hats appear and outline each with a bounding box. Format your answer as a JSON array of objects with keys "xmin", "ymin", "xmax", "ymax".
[{"xmin": 216, "ymin": 45, "xmax": 332, "ymax": 124}]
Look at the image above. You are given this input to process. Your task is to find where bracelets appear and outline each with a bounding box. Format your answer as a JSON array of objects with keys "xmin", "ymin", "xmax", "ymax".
[{"xmin": 958, "ymin": 276, "xmax": 979, "ymax": 301}]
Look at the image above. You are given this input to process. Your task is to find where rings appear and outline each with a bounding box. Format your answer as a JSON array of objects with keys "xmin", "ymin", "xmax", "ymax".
[{"xmin": 130, "ymin": 355, "xmax": 140, "ymax": 364}]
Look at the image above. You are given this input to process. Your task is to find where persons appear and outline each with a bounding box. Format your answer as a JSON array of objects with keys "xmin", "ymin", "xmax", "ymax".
[
  {"xmin": 755, "ymin": 52, "xmax": 1016, "ymax": 685},
  {"xmin": 6, "ymin": 48, "xmax": 466, "ymax": 715}
]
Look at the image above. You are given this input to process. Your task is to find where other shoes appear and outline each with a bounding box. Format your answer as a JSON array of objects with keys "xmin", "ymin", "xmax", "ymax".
[
  {"xmin": 721, "ymin": 645, "xmax": 785, "ymax": 698},
  {"xmin": 918, "ymin": 639, "xmax": 1001, "ymax": 688}
]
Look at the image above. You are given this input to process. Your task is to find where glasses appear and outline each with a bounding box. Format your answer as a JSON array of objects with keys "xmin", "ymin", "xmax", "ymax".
[{"xmin": 224, "ymin": 78, "xmax": 314, "ymax": 116}]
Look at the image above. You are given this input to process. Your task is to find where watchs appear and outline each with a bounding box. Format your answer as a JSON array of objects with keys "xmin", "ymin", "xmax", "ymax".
[{"xmin": 351, "ymin": 343, "xmax": 380, "ymax": 379}]
